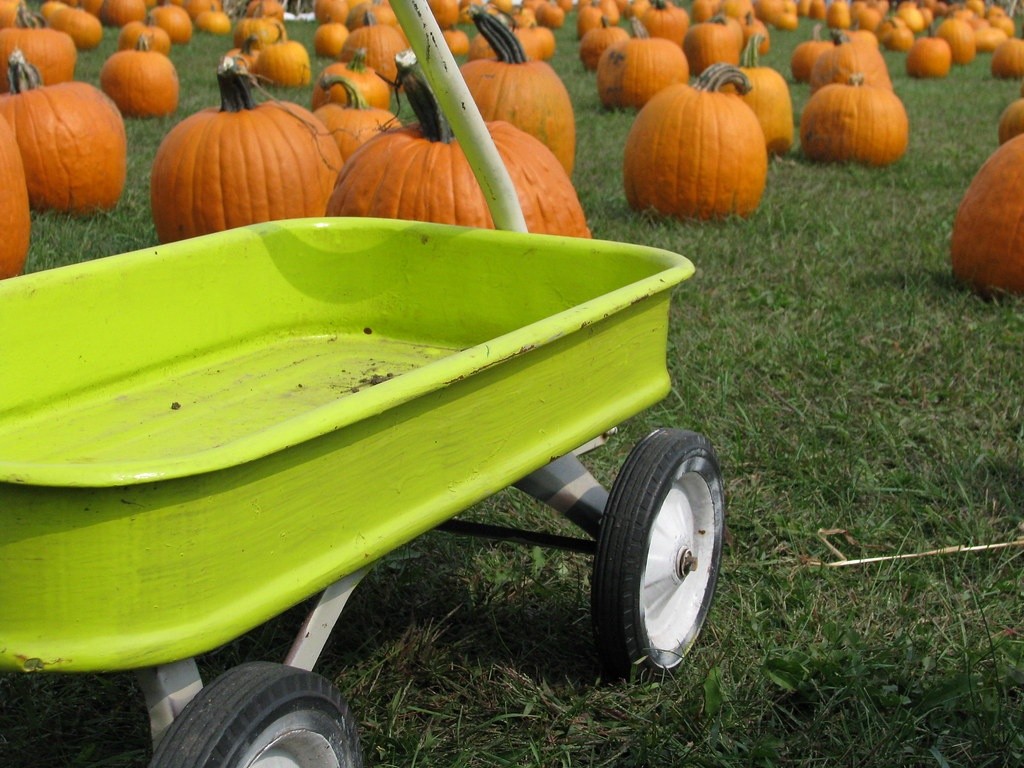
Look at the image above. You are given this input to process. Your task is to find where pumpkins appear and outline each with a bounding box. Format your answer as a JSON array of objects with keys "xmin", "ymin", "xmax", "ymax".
[{"xmin": 0, "ymin": 0, "xmax": 1024, "ymax": 297}]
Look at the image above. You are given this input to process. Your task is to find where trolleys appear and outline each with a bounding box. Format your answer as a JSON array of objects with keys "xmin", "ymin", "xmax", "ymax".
[{"xmin": 1, "ymin": 1, "xmax": 729, "ymax": 766}]
